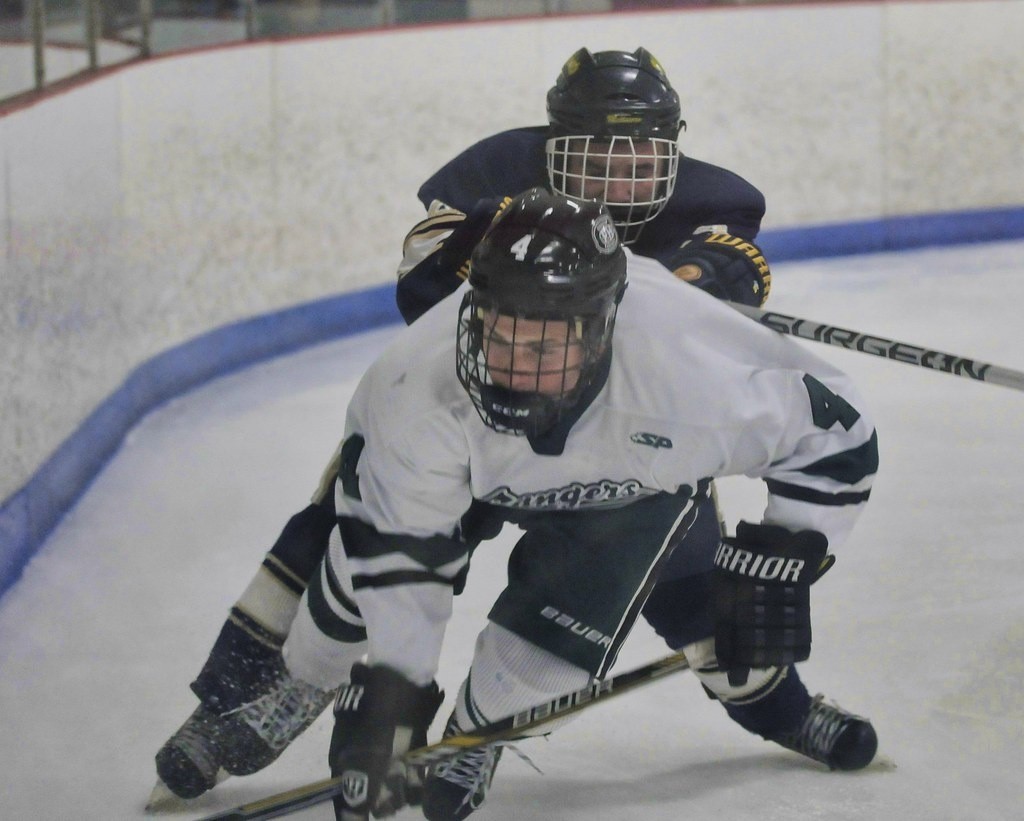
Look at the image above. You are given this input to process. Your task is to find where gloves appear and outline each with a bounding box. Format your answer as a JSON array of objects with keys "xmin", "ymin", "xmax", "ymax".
[
  {"xmin": 712, "ymin": 520, "xmax": 836, "ymax": 687},
  {"xmin": 327, "ymin": 660, "xmax": 448, "ymax": 821},
  {"xmin": 438, "ymin": 193, "xmax": 515, "ymax": 281},
  {"xmin": 658, "ymin": 224, "xmax": 774, "ymax": 310}
]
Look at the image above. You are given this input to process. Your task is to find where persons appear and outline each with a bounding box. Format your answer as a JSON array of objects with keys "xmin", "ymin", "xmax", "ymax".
[{"xmin": 146, "ymin": 45, "xmax": 896, "ymax": 821}]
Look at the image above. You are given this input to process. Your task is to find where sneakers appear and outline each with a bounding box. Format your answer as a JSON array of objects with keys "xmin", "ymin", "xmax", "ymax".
[
  {"xmin": 772, "ymin": 692, "xmax": 898, "ymax": 772},
  {"xmin": 419, "ymin": 706, "xmax": 504, "ymax": 821},
  {"xmin": 141, "ymin": 701, "xmax": 233, "ymax": 815},
  {"xmin": 206, "ymin": 647, "xmax": 346, "ymax": 785}
]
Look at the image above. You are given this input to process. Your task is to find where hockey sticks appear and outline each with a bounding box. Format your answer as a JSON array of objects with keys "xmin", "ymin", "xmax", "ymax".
[
  {"xmin": 723, "ymin": 299, "xmax": 1024, "ymax": 393},
  {"xmin": 199, "ymin": 651, "xmax": 688, "ymax": 821}
]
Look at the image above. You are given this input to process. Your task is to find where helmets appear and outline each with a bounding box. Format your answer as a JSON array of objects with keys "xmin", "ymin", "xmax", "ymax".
[
  {"xmin": 454, "ymin": 186, "xmax": 630, "ymax": 437},
  {"xmin": 544, "ymin": 46, "xmax": 687, "ymax": 245}
]
